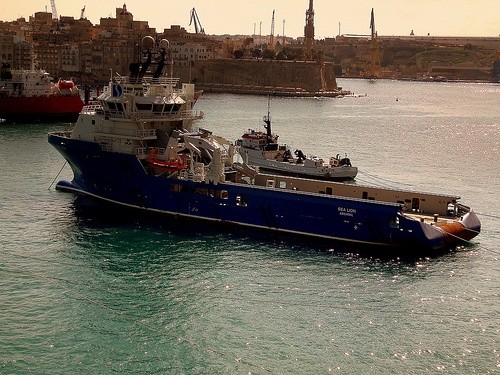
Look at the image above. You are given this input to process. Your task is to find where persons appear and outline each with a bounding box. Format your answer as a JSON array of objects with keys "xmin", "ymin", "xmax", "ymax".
[
  {"xmin": 294, "ymin": 148, "xmax": 307, "ymax": 162},
  {"xmin": 282, "ymin": 149, "xmax": 293, "ymax": 163}
]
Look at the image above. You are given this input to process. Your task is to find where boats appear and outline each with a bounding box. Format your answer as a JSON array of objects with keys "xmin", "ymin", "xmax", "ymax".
[
  {"xmin": 48, "ymin": 33, "xmax": 482, "ymax": 264},
  {"xmin": 0, "ymin": 51, "xmax": 85, "ymax": 118}
]
